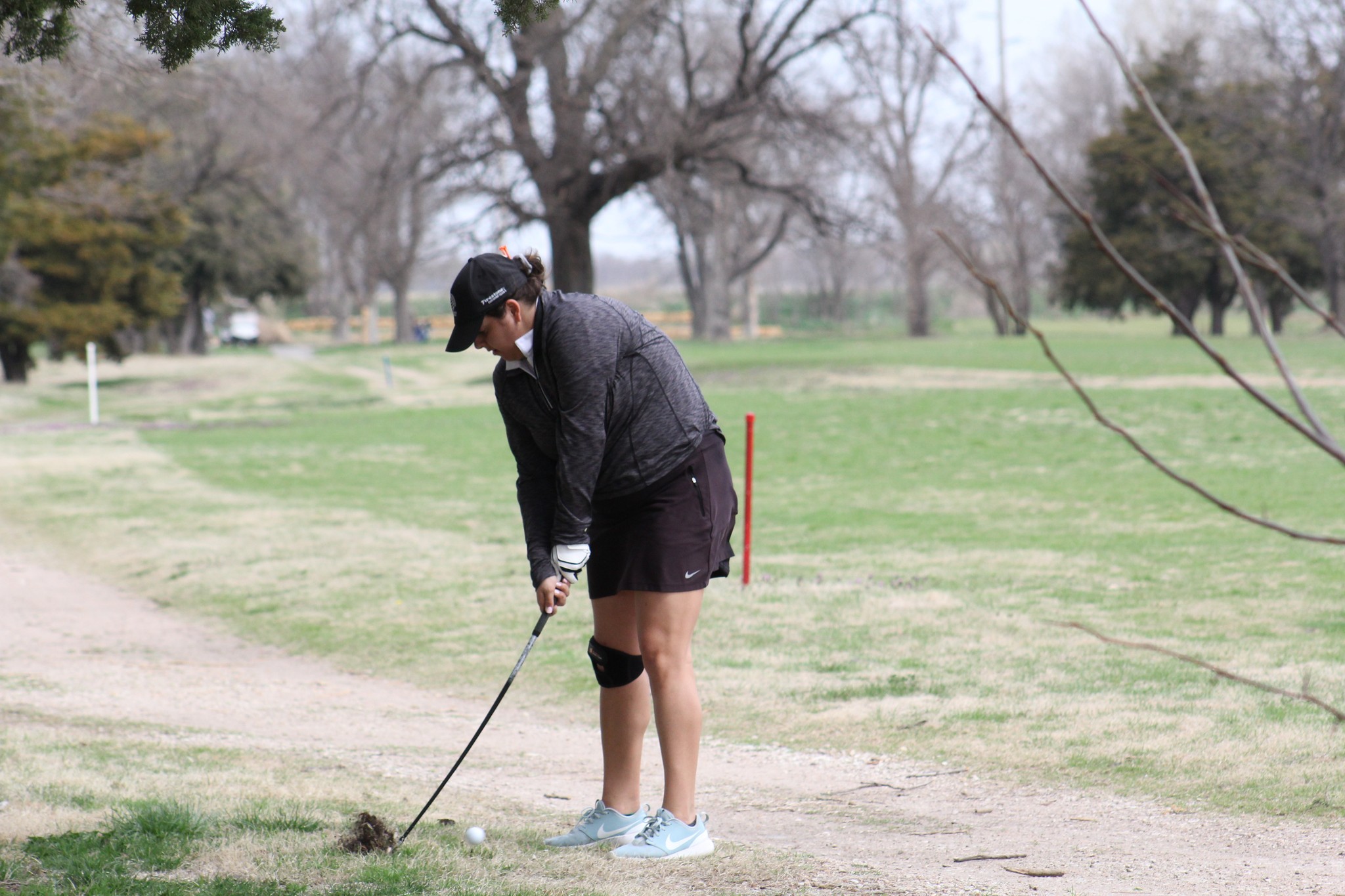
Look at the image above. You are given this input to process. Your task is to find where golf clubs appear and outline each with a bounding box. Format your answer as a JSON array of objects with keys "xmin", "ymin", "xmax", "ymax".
[{"xmin": 358, "ymin": 572, "xmax": 574, "ymax": 855}]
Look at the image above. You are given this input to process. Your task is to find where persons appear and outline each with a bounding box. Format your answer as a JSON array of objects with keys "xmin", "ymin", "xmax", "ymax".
[{"xmin": 445, "ymin": 245, "xmax": 739, "ymax": 860}]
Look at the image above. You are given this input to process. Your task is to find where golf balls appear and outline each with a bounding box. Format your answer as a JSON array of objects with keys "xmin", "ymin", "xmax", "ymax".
[{"xmin": 466, "ymin": 827, "xmax": 485, "ymax": 847}]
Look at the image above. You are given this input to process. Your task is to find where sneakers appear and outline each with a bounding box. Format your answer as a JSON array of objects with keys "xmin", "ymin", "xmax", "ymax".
[
  {"xmin": 543, "ymin": 799, "xmax": 650, "ymax": 850},
  {"xmin": 607, "ymin": 808, "xmax": 715, "ymax": 861}
]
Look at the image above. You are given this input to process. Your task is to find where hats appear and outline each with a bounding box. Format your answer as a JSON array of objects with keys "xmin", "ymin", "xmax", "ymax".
[{"xmin": 445, "ymin": 253, "xmax": 527, "ymax": 352}]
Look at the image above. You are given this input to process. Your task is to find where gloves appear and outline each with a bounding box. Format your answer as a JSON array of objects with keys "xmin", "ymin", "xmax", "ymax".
[{"xmin": 550, "ymin": 544, "xmax": 591, "ymax": 584}]
